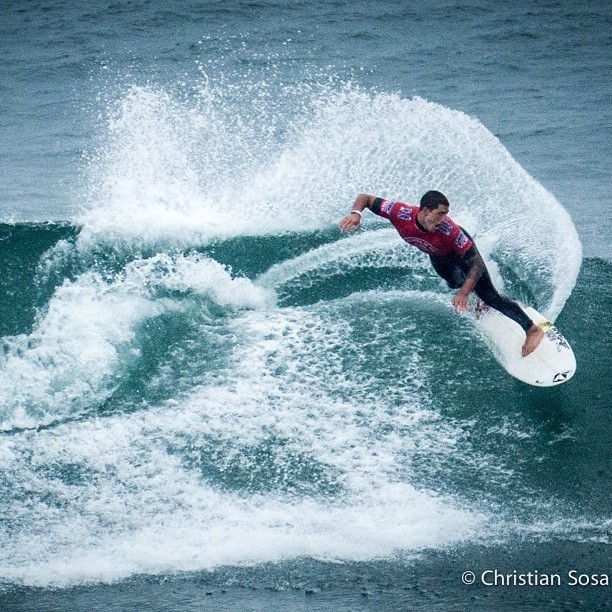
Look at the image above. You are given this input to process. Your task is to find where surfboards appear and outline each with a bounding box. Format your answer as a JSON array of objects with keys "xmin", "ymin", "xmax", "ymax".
[{"xmin": 475, "ymin": 301, "xmax": 577, "ymax": 387}]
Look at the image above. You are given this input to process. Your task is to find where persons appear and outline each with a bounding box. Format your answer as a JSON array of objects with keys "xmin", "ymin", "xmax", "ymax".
[{"xmin": 336, "ymin": 189, "xmax": 546, "ymax": 358}]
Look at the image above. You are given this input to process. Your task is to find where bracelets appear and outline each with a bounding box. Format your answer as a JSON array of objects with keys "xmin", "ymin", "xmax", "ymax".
[{"xmin": 350, "ymin": 209, "xmax": 362, "ymax": 218}]
